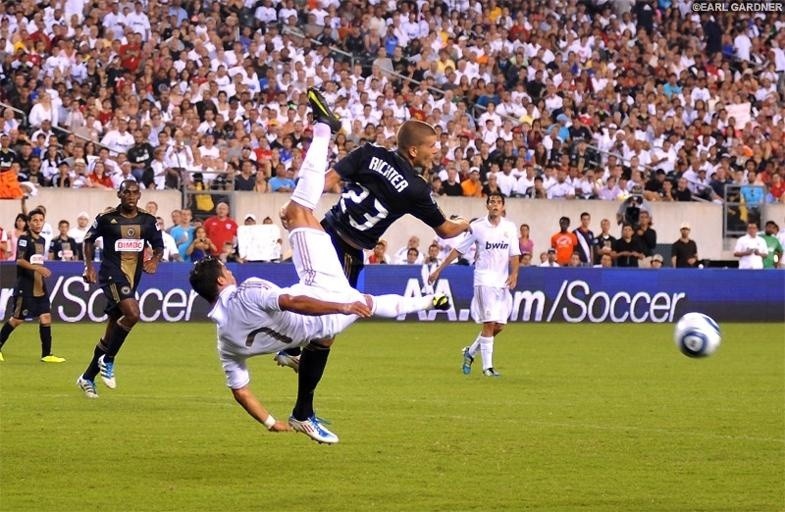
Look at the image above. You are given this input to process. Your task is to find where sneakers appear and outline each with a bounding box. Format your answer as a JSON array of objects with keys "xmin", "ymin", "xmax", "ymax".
[
  {"xmin": 98, "ymin": 354, "xmax": 116, "ymax": 389},
  {"xmin": 288, "ymin": 412, "xmax": 338, "ymax": 446},
  {"xmin": 484, "ymin": 368, "xmax": 501, "ymax": 377},
  {"xmin": 434, "ymin": 293, "xmax": 452, "ymax": 309},
  {"xmin": 0, "ymin": 353, "xmax": 5, "ymax": 362},
  {"xmin": 462, "ymin": 347, "xmax": 474, "ymax": 375},
  {"xmin": 274, "ymin": 351, "xmax": 300, "ymax": 375},
  {"xmin": 76, "ymin": 372, "xmax": 100, "ymax": 399},
  {"xmin": 40, "ymin": 355, "xmax": 66, "ymax": 363},
  {"xmin": 307, "ymin": 88, "xmax": 341, "ymax": 136}
]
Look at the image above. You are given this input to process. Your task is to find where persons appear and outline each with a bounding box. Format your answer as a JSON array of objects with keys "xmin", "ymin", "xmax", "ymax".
[
  {"xmin": 539, "ymin": 248, "xmax": 561, "ymax": 267},
  {"xmin": 262, "ymin": 209, "xmax": 275, "ymax": 224},
  {"xmin": 551, "ymin": 216, "xmax": 578, "ymax": 265},
  {"xmin": 74, "ymin": 178, "xmax": 164, "ymax": 400},
  {"xmin": 520, "ymin": 251, "xmax": 534, "ymax": 267},
  {"xmin": 277, "ymin": 119, "xmax": 474, "ymax": 445},
  {"xmin": 220, "ymin": 240, "xmax": 247, "ymax": 264},
  {"xmin": 651, "ymin": 254, "xmax": 663, "ymax": 268},
  {"xmin": 426, "ymin": 190, "xmax": 524, "ymax": 377},
  {"xmin": 47, "ymin": 220, "xmax": 78, "ymax": 262},
  {"xmin": 774, "ymin": 226, "xmax": 785, "ymax": 267},
  {"xmin": 66, "ymin": 211, "xmax": 99, "ymax": 244},
  {"xmin": 186, "ymin": 225, "xmax": 217, "ymax": 263},
  {"xmin": 571, "ymin": 211, "xmax": 595, "ymax": 263},
  {"xmin": 368, "ymin": 242, "xmax": 388, "ymax": 265},
  {"xmin": 422, "ymin": 244, "xmax": 443, "ymax": 265},
  {"xmin": 171, "ymin": 207, "xmax": 201, "ymax": 258},
  {"xmin": 315, "ymin": 0, "xmax": 451, "ymax": 194},
  {"xmin": 202, "ymin": 200, "xmax": 239, "ymax": 255},
  {"xmin": 570, "ymin": 252, "xmax": 582, "ymax": 266},
  {"xmin": 244, "ymin": 212, "xmax": 260, "ymax": 224},
  {"xmin": 162, "ymin": 284, "xmax": 164, "ymax": 295},
  {"xmin": 378, "ymin": 115, "xmax": 384, "ymax": 115},
  {"xmin": 669, "ymin": 225, "xmax": 700, "ymax": 270},
  {"xmin": 616, "ymin": 186, "xmax": 652, "ymax": 230},
  {"xmin": 153, "ymin": 216, "xmax": 182, "ymax": 261},
  {"xmin": 21, "ymin": 194, "xmax": 52, "ymax": 259},
  {"xmin": 732, "ymin": 219, "xmax": 770, "ymax": 268},
  {"xmin": 1, "ymin": 0, "xmax": 187, "ymax": 198},
  {"xmin": 188, "ymin": 0, "xmax": 315, "ymax": 191},
  {"xmin": 758, "ymin": 221, "xmax": 783, "ymax": 269},
  {"xmin": 600, "ymin": 253, "xmax": 615, "ymax": 267},
  {"xmin": 611, "ymin": 225, "xmax": 643, "ymax": 267},
  {"xmin": 166, "ymin": 207, "xmax": 182, "ymax": 234},
  {"xmin": 374, "ymin": 115, "xmax": 382, "ymax": 125},
  {"xmin": 519, "ymin": 224, "xmax": 534, "ymax": 253},
  {"xmin": 370, "ymin": 115, "xmax": 374, "ymax": 120},
  {"xmin": 594, "ymin": 219, "xmax": 619, "ymax": 264},
  {"xmin": 0, "ymin": 209, "xmax": 65, "ymax": 364},
  {"xmin": 188, "ymin": 86, "xmax": 451, "ymax": 434},
  {"xmin": 635, "ymin": 211, "xmax": 657, "ymax": 250},
  {"xmin": 7, "ymin": 213, "xmax": 30, "ymax": 262},
  {"xmin": 539, "ymin": 254, "xmax": 547, "ymax": 261},
  {"xmin": 0, "ymin": 227, "xmax": 12, "ymax": 261},
  {"xmin": 164, "ymin": 286, "xmax": 168, "ymax": 287},
  {"xmin": 146, "ymin": 200, "xmax": 155, "ymax": 213},
  {"xmin": 395, "ymin": 235, "xmax": 421, "ymax": 256},
  {"xmin": 401, "ymin": 247, "xmax": 419, "ymax": 264}
]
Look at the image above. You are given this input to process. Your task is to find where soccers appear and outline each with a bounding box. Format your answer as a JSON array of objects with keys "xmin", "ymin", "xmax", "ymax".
[{"xmin": 675, "ymin": 311, "xmax": 721, "ymax": 360}]
